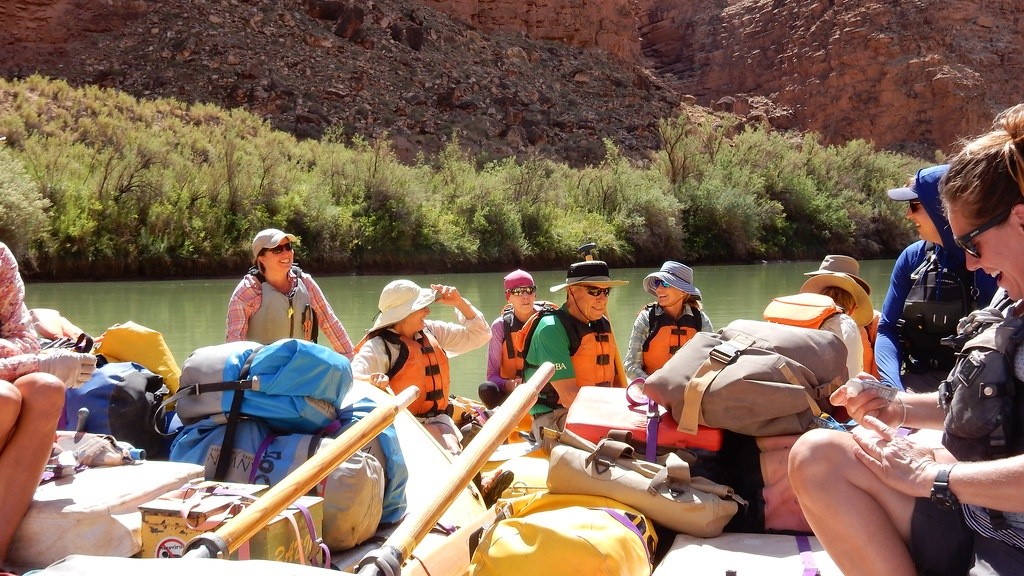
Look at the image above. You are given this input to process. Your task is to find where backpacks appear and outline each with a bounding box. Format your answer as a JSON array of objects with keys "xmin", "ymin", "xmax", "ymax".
[
  {"xmin": 937, "ymin": 295, "xmax": 1024, "ymax": 462},
  {"xmin": 29, "ymin": 293, "xmax": 864, "ymax": 576},
  {"xmin": 899, "ymin": 266, "xmax": 981, "ymax": 372}
]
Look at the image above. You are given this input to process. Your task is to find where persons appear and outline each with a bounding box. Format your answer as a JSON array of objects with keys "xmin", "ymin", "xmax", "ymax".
[
  {"xmin": 226, "ymin": 229, "xmax": 358, "ymax": 357},
  {"xmin": 874, "ymin": 164, "xmax": 999, "ymax": 394},
  {"xmin": 800, "ymin": 255, "xmax": 882, "ymax": 379},
  {"xmin": 787, "ymin": 107, "xmax": 1024, "ymax": 576},
  {"xmin": 0, "ymin": 241, "xmax": 96, "ymax": 576},
  {"xmin": 521, "ymin": 261, "xmax": 630, "ymax": 457},
  {"xmin": 622, "ymin": 261, "xmax": 714, "ymax": 381},
  {"xmin": 349, "ymin": 279, "xmax": 515, "ymax": 508},
  {"xmin": 478, "ymin": 269, "xmax": 558, "ymax": 440}
]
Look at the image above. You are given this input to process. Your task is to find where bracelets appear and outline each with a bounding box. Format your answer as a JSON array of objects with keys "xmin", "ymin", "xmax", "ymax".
[{"xmin": 896, "ymin": 397, "xmax": 906, "ymax": 429}]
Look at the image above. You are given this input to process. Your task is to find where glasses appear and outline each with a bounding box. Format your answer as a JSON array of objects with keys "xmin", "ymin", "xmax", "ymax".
[
  {"xmin": 909, "ymin": 201, "xmax": 922, "ymax": 214},
  {"xmin": 512, "ymin": 285, "xmax": 535, "ymax": 296},
  {"xmin": 954, "ymin": 201, "xmax": 1017, "ymax": 258},
  {"xmin": 587, "ymin": 288, "xmax": 612, "ymax": 296},
  {"xmin": 272, "ymin": 241, "xmax": 294, "ymax": 254},
  {"xmin": 654, "ymin": 281, "xmax": 672, "ymax": 288}
]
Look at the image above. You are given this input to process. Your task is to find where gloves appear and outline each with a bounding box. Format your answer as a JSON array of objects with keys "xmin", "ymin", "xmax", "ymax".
[{"xmin": 39, "ymin": 349, "xmax": 97, "ymax": 389}]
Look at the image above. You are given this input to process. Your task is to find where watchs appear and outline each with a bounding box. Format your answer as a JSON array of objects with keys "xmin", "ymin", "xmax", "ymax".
[{"xmin": 930, "ymin": 463, "xmax": 960, "ymax": 511}]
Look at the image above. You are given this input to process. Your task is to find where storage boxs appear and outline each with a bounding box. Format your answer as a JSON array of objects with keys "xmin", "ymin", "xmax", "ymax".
[
  {"xmin": 564, "ymin": 384, "xmax": 723, "ymax": 456},
  {"xmin": 139, "ymin": 480, "xmax": 323, "ymax": 568}
]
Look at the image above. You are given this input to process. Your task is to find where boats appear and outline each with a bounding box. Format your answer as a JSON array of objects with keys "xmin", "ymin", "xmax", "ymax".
[{"xmin": 446, "ymin": 395, "xmax": 550, "ymax": 501}]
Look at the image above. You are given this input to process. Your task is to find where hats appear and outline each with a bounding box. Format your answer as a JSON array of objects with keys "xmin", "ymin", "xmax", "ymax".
[
  {"xmin": 888, "ymin": 179, "xmax": 918, "ymax": 200},
  {"xmin": 369, "ymin": 279, "xmax": 435, "ymax": 332},
  {"xmin": 803, "ymin": 255, "xmax": 871, "ymax": 295},
  {"xmin": 550, "ymin": 260, "xmax": 629, "ymax": 292},
  {"xmin": 643, "ymin": 261, "xmax": 702, "ymax": 301},
  {"xmin": 254, "ymin": 228, "xmax": 297, "ymax": 258},
  {"xmin": 505, "ymin": 270, "xmax": 534, "ymax": 300},
  {"xmin": 801, "ymin": 273, "xmax": 873, "ymax": 327}
]
